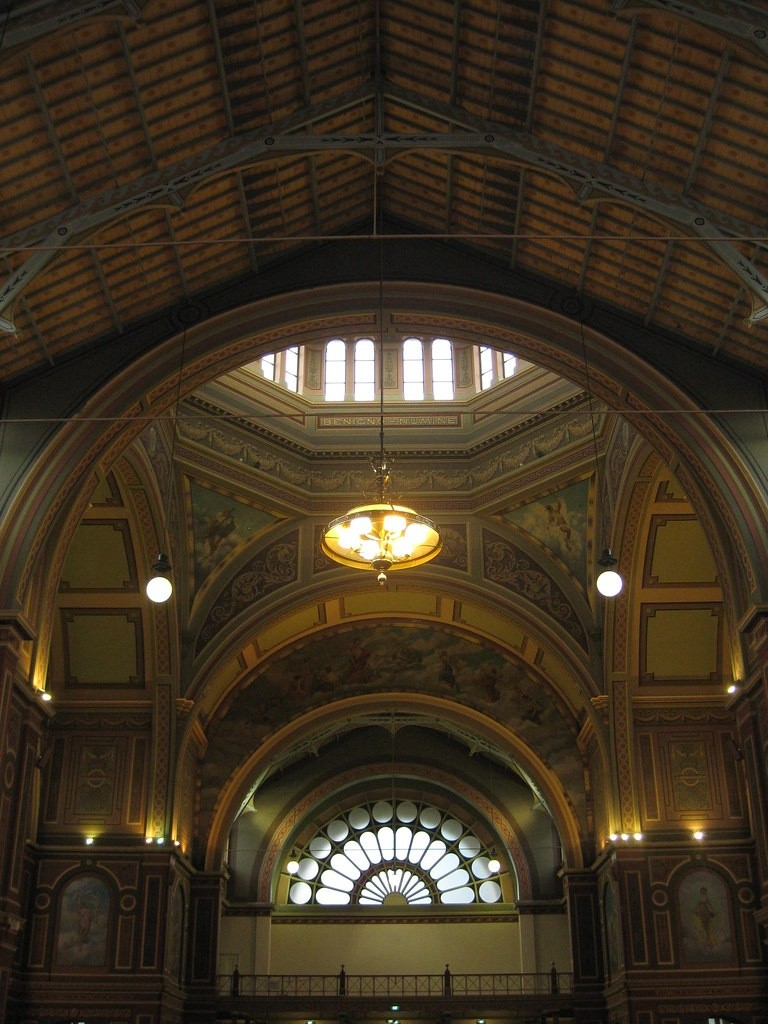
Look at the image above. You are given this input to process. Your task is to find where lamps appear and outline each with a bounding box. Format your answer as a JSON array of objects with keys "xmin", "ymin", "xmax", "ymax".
[
  {"xmin": 488, "ymin": 746, "xmax": 500, "ymax": 872},
  {"xmin": 145, "ymin": 294, "xmax": 192, "ymax": 604},
  {"xmin": 390, "ymin": 732, "xmax": 401, "ymax": 886},
  {"xmin": 579, "ymin": 314, "xmax": 623, "ymax": 598},
  {"xmin": 286, "ymin": 745, "xmax": 300, "ymax": 875},
  {"xmin": 318, "ymin": 57, "xmax": 445, "ymax": 586}
]
